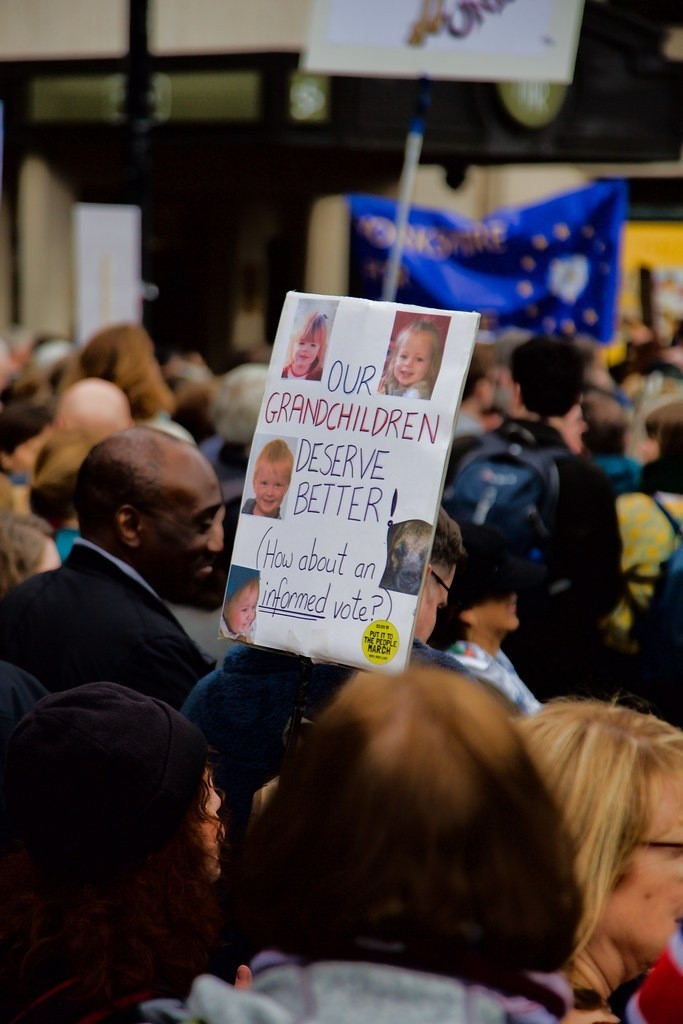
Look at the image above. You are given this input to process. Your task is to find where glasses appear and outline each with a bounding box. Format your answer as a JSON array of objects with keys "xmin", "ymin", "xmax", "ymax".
[{"xmin": 431, "ymin": 571, "xmax": 463, "ymax": 625}]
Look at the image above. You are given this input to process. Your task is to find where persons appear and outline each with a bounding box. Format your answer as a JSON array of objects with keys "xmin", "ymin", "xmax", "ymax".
[
  {"xmin": 220, "ymin": 564, "xmax": 260, "ymax": 644},
  {"xmin": 281, "ymin": 313, "xmax": 327, "ymax": 381},
  {"xmin": 241, "ymin": 439, "xmax": 294, "ymax": 520},
  {"xmin": 379, "ymin": 320, "xmax": 438, "ymax": 400},
  {"xmin": 139, "ymin": 669, "xmax": 583, "ymax": 1024},
  {"xmin": 1, "ymin": 320, "xmax": 683, "ymax": 1024},
  {"xmin": 515, "ymin": 702, "xmax": 683, "ymax": 1024}
]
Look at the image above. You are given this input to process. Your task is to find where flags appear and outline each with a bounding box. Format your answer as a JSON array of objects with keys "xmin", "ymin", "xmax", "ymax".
[{"xmin": 351, "ymin": 180, "xmax": 625, "ymax": 342}]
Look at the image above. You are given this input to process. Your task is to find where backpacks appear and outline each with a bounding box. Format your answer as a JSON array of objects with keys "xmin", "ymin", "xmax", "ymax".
[
  {"xmin": 441, "ymin": 430, "xmax": 580, "ymax": 561},
  {"xmin": 624, "ymin": 498, "xmax": 683, "ymax": 720}
]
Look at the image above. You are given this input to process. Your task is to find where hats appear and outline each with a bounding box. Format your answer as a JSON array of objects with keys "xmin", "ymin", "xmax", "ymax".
[
  {"xmin": 0, "ymin": 681, "xmax": 201, "ymax": 871},
  {"xmin": 456, "ymin": 519, "xmax": 547, "ymax": 595}
]
